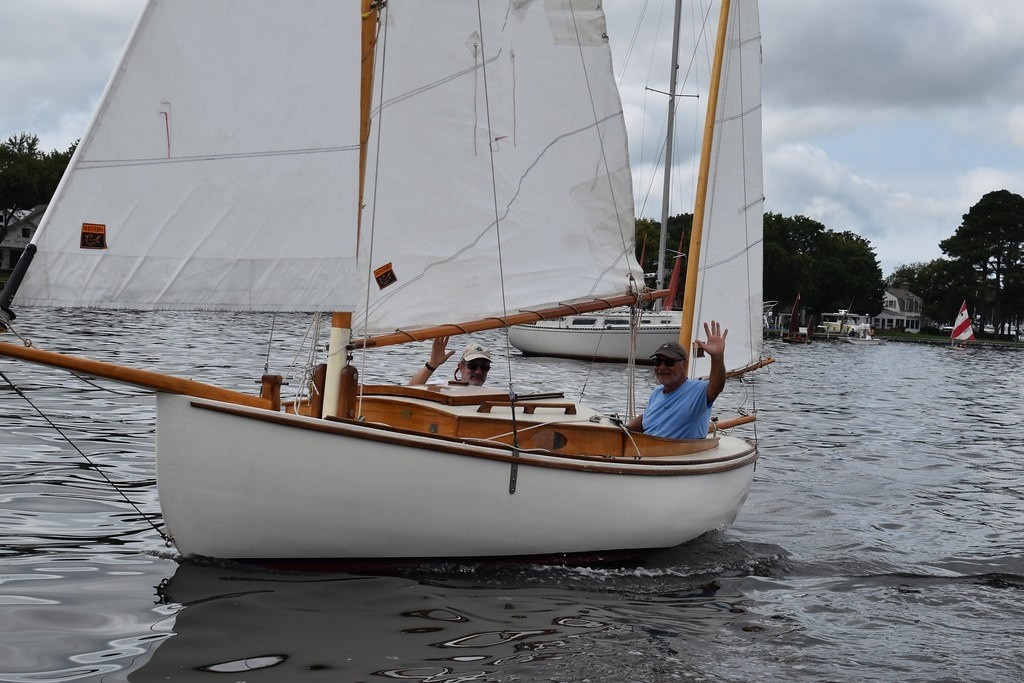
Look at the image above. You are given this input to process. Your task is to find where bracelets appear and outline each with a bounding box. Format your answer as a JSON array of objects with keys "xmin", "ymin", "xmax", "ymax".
[{"xmin": 425, "ymin": 362, "xmax": 436, "ymax": 372}]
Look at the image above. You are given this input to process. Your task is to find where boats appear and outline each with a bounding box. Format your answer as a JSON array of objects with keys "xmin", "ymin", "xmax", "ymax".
[
  {"xmin": 839, "ymin": 337, "xmax": 887, "ymax": 346},
  {"xmin": 782, "ymin": 336, "xmax": 813, "ymax": 343}
]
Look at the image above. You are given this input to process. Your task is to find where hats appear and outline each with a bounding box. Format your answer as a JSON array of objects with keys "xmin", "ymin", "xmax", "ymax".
[
  {"xmin": 649, "ymin": 342, "xmax": 687, "ymax": 360},
  {"xmin": 462, "ymin": 344, "xmax": 493, "ymax": 363}
]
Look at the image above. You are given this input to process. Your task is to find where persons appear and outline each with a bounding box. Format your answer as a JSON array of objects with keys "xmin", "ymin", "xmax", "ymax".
[
  {"xmin": 406, "ymin": 335, "xmax": 494, "ymax": 386},
  {"xmin": 956, "ymin": 342, "xmax": 961, "ymax": 347},
  {"xmin": 869, "ymin": 327, "xmax": 874, "ymax": 337},
  {"xmin": 849, "ymin": 328, "xmax": 855, "ymax": 337},
  {"xmin": 626, "ymin": 320, "xmax": 728, "ymax": 438},
  {"xmin": 962, "ymin": 342, "xmax": 967, "ymax": 348}
]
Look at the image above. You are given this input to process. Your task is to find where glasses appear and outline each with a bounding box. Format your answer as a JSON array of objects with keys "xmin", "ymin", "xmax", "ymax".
[
  {"xmin": 652, "ymin": 357, "xmax": 685, "ymax": 367},
  {"xmin": 468, "ymin": 362, "xmax": 490, "ymax": 371}
]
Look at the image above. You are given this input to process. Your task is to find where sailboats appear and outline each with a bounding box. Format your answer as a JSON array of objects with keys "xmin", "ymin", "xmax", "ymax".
[
  {"xmin": 0, "ymin": 0, "xmax": 775, "ymax": 562},
  {"xmin": 507, "ymin": 0, "xmax": 710, "ymax": 363},
  {"xmin": 945, "ymin": 300, "xmax": 974, "ymax": 349}
]
